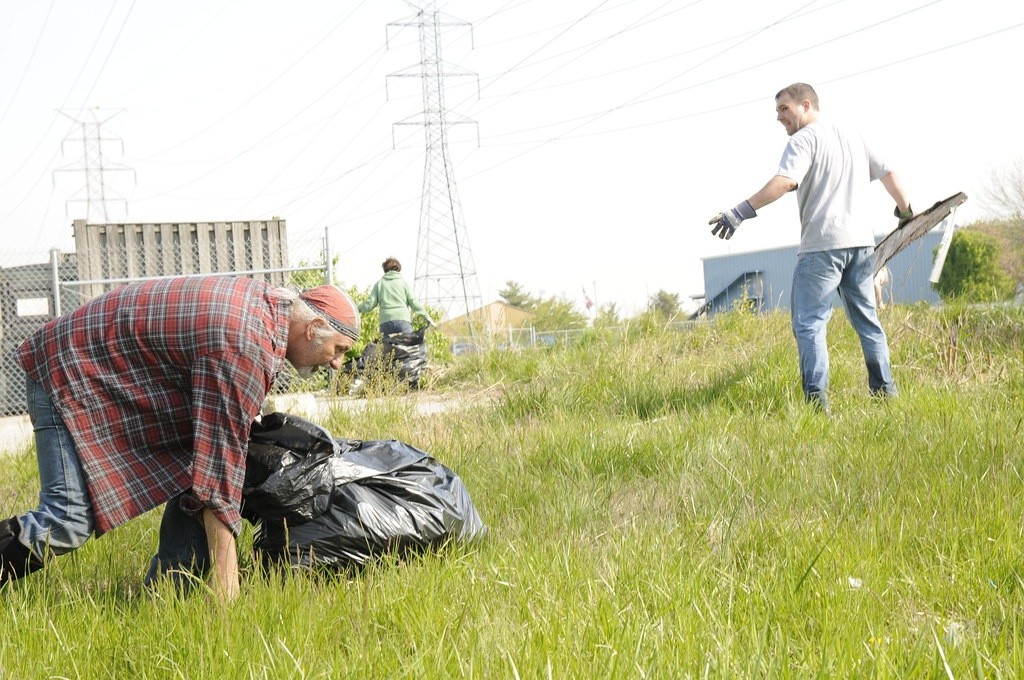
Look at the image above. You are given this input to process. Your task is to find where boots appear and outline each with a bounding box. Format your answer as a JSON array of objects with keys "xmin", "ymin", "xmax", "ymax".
[{"xmin": 0, "ymin": 513, "xmax": 44, "ymax": 585}]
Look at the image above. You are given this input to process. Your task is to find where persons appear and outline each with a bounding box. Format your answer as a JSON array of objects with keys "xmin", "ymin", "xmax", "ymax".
[
  {"xmin": 0, "ymin": 275, "xmax": 360, "ymax": 602},
  {"xmin": 708, "ymin": 82, "xmax": 914, "ymax": 414},
  {"xmin": 356, "ymin": 259, "xmax": 436, "ymax": 377}
]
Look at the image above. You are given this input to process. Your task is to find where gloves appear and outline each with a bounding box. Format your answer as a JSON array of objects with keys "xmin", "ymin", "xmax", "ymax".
[
  {"xmin": 707, "ymin": 200, "xmax": 757, "ymax": 240},
  {"xmin": 893, "ymin": 203, "xmax": 914, "ymax": 228}
]
